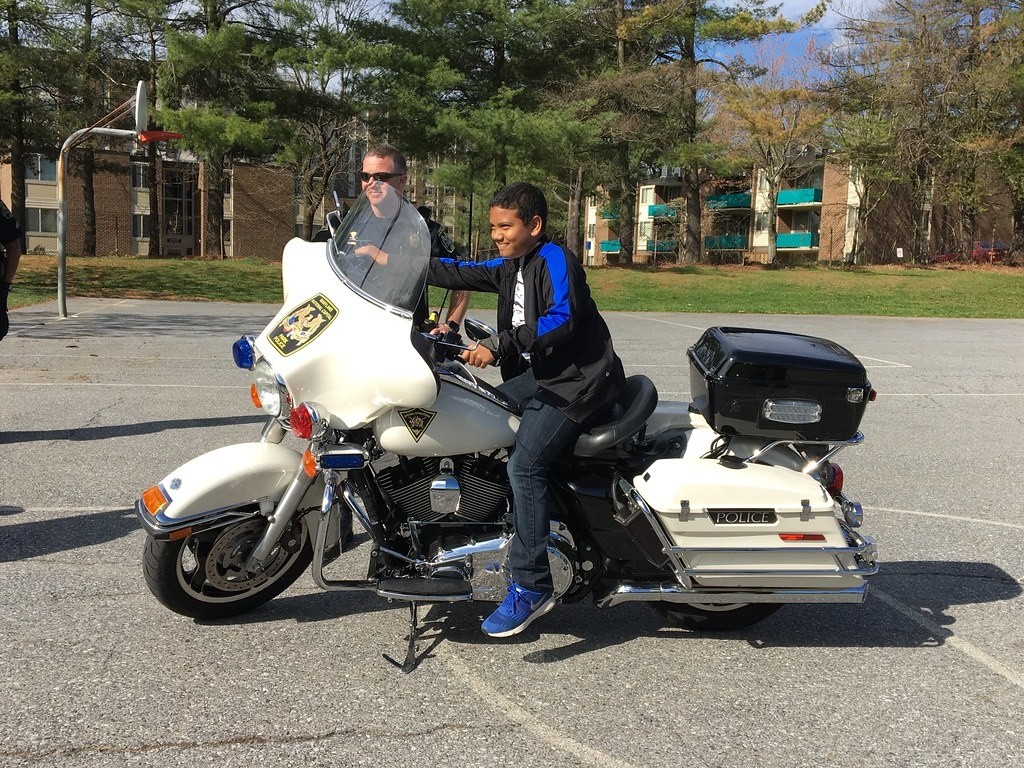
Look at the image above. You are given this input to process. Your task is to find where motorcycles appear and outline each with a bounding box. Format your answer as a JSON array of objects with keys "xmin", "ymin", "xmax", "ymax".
[{"xmin": 135, "ymin": 178, "xmax": 879, "ymax": 674}]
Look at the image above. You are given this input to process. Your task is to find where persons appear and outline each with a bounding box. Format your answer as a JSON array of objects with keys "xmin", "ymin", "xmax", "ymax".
[
  {"xmin": 354, "ymin": 181, "xmax": 614, "ymax": 638},
  {"xmin": 314, "ymin": 144, "xmax": 470, "ymax": 568},
  {"xmin": 0, "ymin": 200, "xmax": 21, "ymax": 342}
]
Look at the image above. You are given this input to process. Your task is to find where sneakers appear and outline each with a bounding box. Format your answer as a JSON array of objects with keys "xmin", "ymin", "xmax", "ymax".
[{"xmin": 481, "ymin": 576, "xmax": 555, "ymax": 637}]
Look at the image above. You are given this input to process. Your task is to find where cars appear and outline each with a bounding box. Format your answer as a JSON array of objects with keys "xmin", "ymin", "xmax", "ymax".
[{"xmin": 931, "ymin": 241, "xmax": 1011, "ymax": 265}]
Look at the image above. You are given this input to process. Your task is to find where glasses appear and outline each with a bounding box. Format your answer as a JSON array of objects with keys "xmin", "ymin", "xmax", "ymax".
[{"xmin": 361, "ymin": 171, "xmax": 404, "ymax": 182}]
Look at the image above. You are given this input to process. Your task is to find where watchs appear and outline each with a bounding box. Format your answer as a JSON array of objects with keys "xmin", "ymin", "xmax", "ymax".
[
  {"xmin": 4, "ymin": 282, "xmax": 13, "ymax": 291},
  {"xmin": 443, "ymin": 320, "xmax": 459, "ymax": 332}
]
